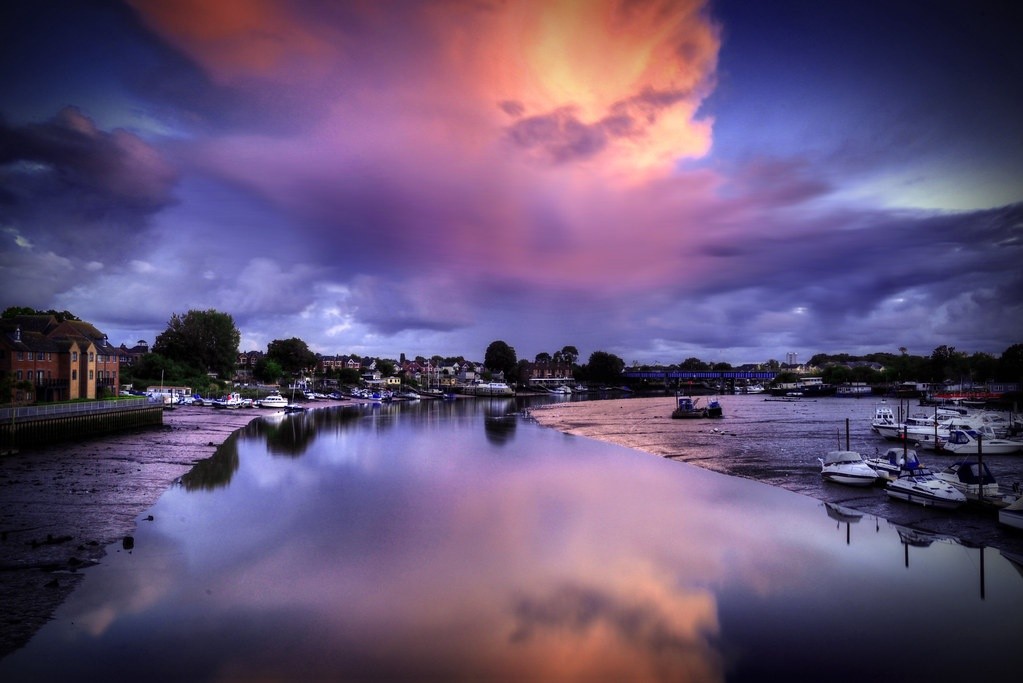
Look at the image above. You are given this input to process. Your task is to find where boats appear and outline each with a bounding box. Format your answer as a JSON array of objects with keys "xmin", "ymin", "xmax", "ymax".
[
  {"xmin": 884, "ymin": 465, "xmax": 968, "ymax": 510},
  {"xmin": 261, "ymin": 390, "xmax": 288, "ymax": 408},
  {"xmin": 461, "ymin": 382, "xmax": 515, "ymax": 398},
  {"xmin": 552, "ymin": 384, "xmax": 588, "ymax": 394},
  {"xmin": 931, "ymin": 461, "xmax": 1000, "ymax": 498},
  {"xmin": 998, "ymin": 495, "xmax": 1023, "ymax": 529},
  {"xmin": 734, "ymin": 379, "xmax": 765, "ymax": 395},
  {"xmin": 119, "ymin": 370, "xmax": 264, "ymax": 409},
  {"xmin": 672, "ymin": 388, "xmax": 722, "ymax": 418},
  {"xmin": 302, "ymin": 382, "xmax": 420, "ymax": 401},
  {"xmin": 817, "ymin": 450, "xmax": 879, "ymax": 487},
  {"xmin": 870, "ymin": 397, "xmax": 1023, "ymax": 456},
  {"xmin": 863, "ymin": 448, "xmax": 927, "ymax": 482}
]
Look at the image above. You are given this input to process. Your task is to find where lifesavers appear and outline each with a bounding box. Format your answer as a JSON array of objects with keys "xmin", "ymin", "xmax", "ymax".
[{"xmin": 937, "ymin": 437, "xmax": 941, "ymax": 444}]
